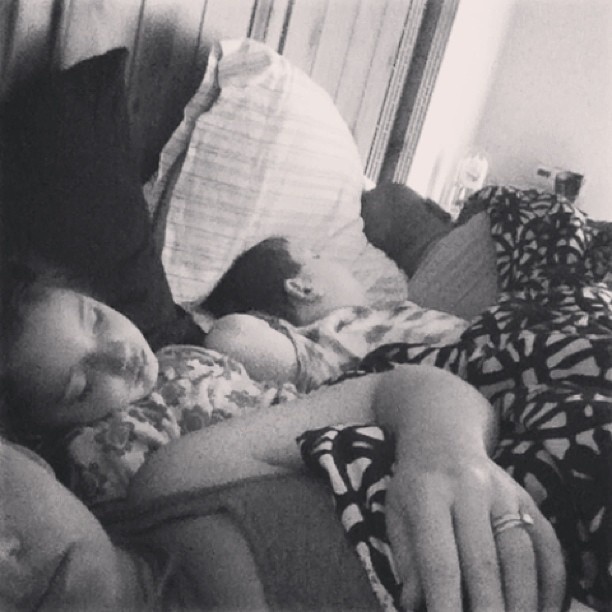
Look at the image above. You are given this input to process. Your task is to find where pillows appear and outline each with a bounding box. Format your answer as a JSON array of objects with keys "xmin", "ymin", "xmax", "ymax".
[
  {"xmin": 150, "ymin": 36, "xmax": 407, "ymax": 315},
  {"xmin": 0, "ymin": 47, "xmax": 205, "ymax": 347}
]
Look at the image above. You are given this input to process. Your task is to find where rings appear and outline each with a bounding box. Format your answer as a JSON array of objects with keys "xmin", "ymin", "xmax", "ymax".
[{"xmin": 493, "ymin": 510, "xmax": 534, "ymax": 533}]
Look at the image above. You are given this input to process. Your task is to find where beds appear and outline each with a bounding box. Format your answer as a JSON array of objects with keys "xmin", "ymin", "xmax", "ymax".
[{"xmin": 0, "ymin": 0, "xmax": 607, "ymax": 611}]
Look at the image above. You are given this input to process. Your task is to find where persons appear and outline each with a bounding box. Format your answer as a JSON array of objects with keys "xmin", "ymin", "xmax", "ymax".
[
  {"xmin": 362, "ymin": 182, "xmax": 498, "ymax": 319},
  {"xmin": 1, "ymin": 362, "xmax": 567, "ymax": 612},
  {"xmin": 0, "ymin": 274, "xmax": 304, "ymax": 512},
  {"xmin": 205, "ymin": 235, "xmax": 472, "ymax": 391}
]
